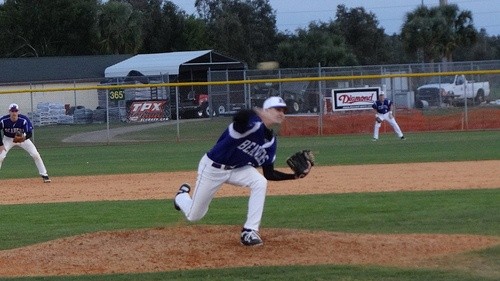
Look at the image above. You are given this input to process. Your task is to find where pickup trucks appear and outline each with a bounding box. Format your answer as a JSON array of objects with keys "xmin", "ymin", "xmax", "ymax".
[{"xmin": 415, "ymin": 75, "xmax": 489, "ymax": 108}]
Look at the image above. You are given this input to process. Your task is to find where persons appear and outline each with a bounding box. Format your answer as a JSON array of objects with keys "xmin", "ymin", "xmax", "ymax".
[
  {"xmin": 371, "ymin": 94, "xmax": 405, "ymax": 140},
  {"xmin": 0, "ymin": 103, "xmax": 52, "ymax": 183},
  {"xmin": 173, "ymin": 95, "xmax": 313, "ymax": 247}
]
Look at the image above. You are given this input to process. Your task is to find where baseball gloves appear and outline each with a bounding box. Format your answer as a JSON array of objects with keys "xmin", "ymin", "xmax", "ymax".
[
  {"xmin": 286, "ymin": 149, "xmax": 315, "ymax": 176},
  {"xmin": 13, "ymin": 132, "xmax": 25, "ymax": 143},
  {"xmin": 376, "ymin": 117, "xmax": 383, "ymax": 123}
]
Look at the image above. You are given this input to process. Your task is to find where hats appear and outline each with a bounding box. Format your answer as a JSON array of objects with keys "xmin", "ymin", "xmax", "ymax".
[
  {"xmin": 9, "ymin": 103, "xmax": 20, "ymax": 112},
  {"xmin": 262, "ymin": 96, "xmax": 287, "ymax": 111},
  {"xmin": 379, "ymin": 91, "xmax": 384, "ymax": 96}
]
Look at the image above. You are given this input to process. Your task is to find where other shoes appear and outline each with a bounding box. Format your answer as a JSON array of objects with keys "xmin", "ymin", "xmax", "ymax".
[
  {"xmin": 174, "ymin": 183, "xmax": 191, "ymax": 211},
  {"xmin": 401, "ymin": 136, "xmax": 405, "ymax": 139},
  {"xmin": 240, "ymin": 230, "xmax": 263, "ymax": 248},
  {"xmin": 372, "ymin": 138, "xmax": 376, "ymax": 141},
  {"xmin": 41, "ymin": 175, "xmax": 51, "ymax": 183}
]
